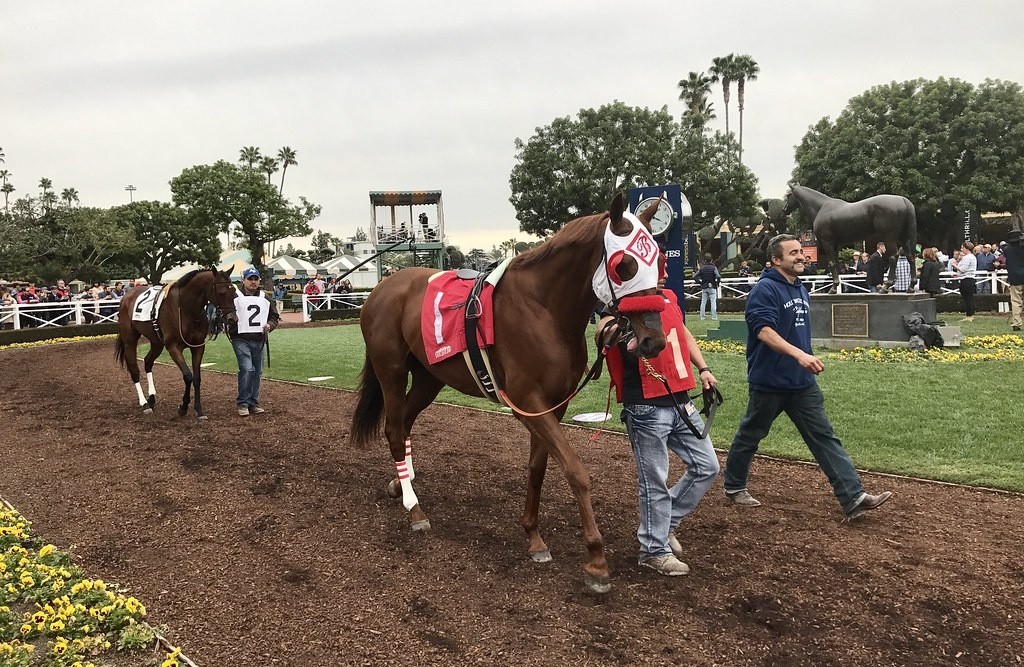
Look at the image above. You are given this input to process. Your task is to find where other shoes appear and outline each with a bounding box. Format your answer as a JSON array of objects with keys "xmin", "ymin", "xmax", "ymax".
[
  {"xmin": 958, "ymin": 317, "xmax": 973, "ymax": 323},
  {"xmin": 1012, "ymin": 325, "xmax": 1020, "ymax": 331}
]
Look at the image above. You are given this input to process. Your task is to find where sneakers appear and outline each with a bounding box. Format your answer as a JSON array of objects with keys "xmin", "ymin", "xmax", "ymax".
[
  {"xmin": 637, "ymin": 551, "xmax": 691, "ymax": 577},
  {"xmin": 237, "ymin": 405, "xmax": 250, "ymax": 416},
  {"xmin": 249, "ymin": 404, "xmax": 265, "ymax": 414},
  {"xmin": 666, "ymin": 531, "xmax": 684, "ymax": 558}
]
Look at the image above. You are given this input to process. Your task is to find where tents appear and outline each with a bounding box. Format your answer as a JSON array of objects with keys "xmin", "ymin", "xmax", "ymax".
[
  {"xmin": 264, "ymin": 254, "xmax": 328, "ymax": 291},
  {"xmin": 318, "ymin": 254, "xmax": 392, "ymax": 289}
]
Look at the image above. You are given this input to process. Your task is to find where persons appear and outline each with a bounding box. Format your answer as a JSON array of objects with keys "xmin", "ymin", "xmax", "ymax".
[
  {"xmin": 420, "ymin": 213, "xmax": 428, "ymax": 242},
  {"xmin": 951, "ymin": 242, "xmax": 977, "ymax": 322},
  {"xmin": 766, "ymin": 260, "xmax": 772, "ymax": 268},
  {"xmin": 798, "ymin": 241, "xmax": 1024, "ymax": 298},
  {"xmin": 0, "ymin": 278, "xmax": 135, "ymax": 330},
  {"xmin": 1003, "ymin": 230, "xmax": 1024, "ymax": 331},
  {"xmin": 226, "ymin": 268, "xmax": 280, "ymax": 415},
  {"xmin": 723, "ymin": 233, "xmax": 892, "ymax": 519},
  {"xmin": 735, "ymin": 260, "xmax": 753, "ymax": 297},
  {"xmin": 272, "ymin": 283, "xmax": 287, "ymax": 321},
  {"xmin": 594, "ymin": 262, "xmax": 719, "ymax": 575},
  {"xmin": 694, "ymin": 253, "xmax": 722, "ymax": 321},
  {"xmin": 303, "ymin": 272, "xmax": 354, "ymax": 314}
]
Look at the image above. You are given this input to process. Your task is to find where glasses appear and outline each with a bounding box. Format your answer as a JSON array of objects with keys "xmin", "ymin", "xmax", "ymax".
[{"xmin": 246, "ymin": 276, "xmax": 259, "ymax": 282}]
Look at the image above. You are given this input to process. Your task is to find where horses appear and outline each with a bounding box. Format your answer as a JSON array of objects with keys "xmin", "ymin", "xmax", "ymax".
[
  {"xmin": 114, "ymin": 265, "xmax": 240, "ymax": 420},
  {"xmin": 781, "ymin": 180, "xmax": 919, "ymax": 293},
  {"xmin": 347, "ymin": 190, "xmax": 667, "ymax": 594}
]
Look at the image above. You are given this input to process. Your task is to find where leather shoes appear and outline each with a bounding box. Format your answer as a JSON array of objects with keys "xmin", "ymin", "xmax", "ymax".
[
  {"xmin": 848, "ymin": 491, "xmax": 892, "ymax": 520},
  {"xmin": 725, "ymin": 491, "xmax": 762, "ymax": 508}
]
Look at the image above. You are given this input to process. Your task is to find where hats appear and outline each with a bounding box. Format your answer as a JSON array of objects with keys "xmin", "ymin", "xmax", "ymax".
[
  {"xmin": 29, "ymin": 289, "xmax": 35, "ymax": 295},
  {"xmin": 308, "ymin": 278, "xmax": 314, "ymax": 284},
  {"xmin": 277, "ymin": 283, "xmax": 283, "ymax": 288},
  {"xmin": 243, "ymin": 268, "xmax": 261, "ymax": 278},
  {"xmin": 852, "ymin": 251, "xmax": 861, "ymax": 256},
  {"xmin": 999, "ymin": 241, "xmax": 1007, "ymax": 246},
  {"xmin": 861, "ymin": 252, "xmax": 869, "ymax": 257},
  {"xmin": 1007, "ymin": 229, "xmax": 1022, "ymax": 243}
]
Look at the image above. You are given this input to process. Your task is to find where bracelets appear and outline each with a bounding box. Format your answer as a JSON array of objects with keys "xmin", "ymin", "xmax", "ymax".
[{"xmin": 699, "ymin": 367, "xmax": 712, "ymax": 374}]
